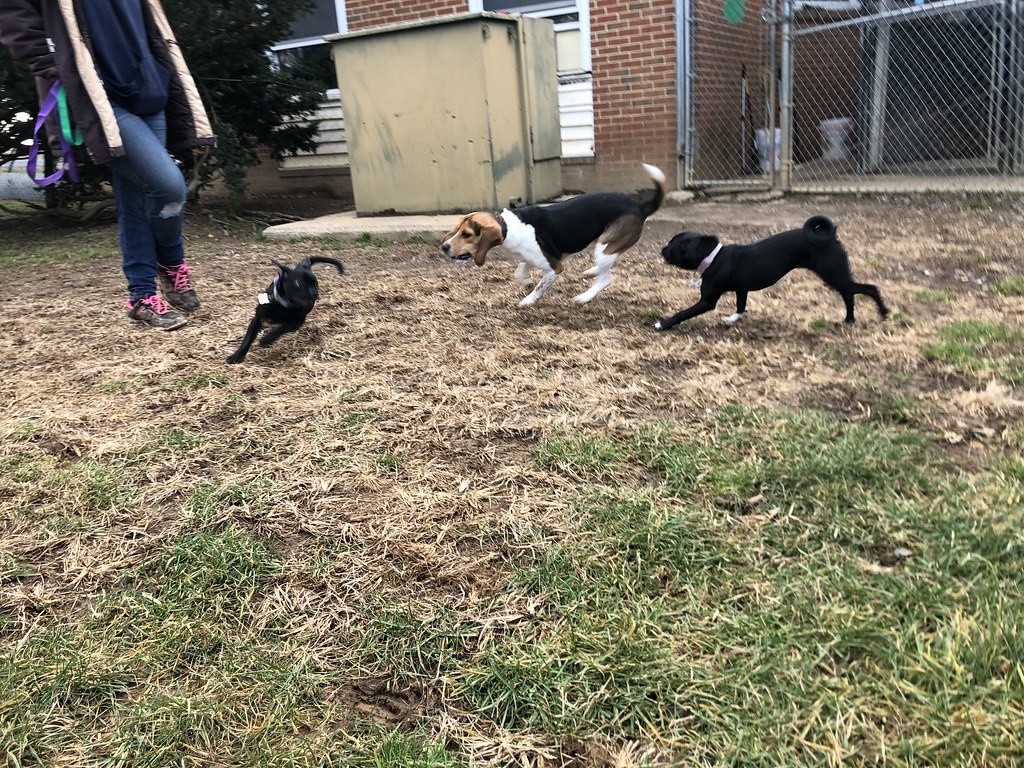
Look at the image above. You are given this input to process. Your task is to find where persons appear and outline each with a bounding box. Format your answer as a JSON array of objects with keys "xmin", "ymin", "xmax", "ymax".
[{"xmin": 0, "ymin": 0, "xmax": 218, "ymax": 330}]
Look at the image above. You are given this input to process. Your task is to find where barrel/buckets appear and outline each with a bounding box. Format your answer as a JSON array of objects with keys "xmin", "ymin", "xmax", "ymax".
[
  {"xmin": 754, "ymin": 128, "xmax": 781, "ymax": 171},
  {"xmin": 819, "ymin": 117, "xmax": 852, "ymax": 160}
]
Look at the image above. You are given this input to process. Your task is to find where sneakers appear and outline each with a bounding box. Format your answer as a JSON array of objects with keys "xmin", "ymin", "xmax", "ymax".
[
  {"xmin": 126, "ymin": 292, "xmax": 188, "ymax": 330},
  {"xmin": 155, "ymin": 259, "xmax": 201, "ymax": 314}
]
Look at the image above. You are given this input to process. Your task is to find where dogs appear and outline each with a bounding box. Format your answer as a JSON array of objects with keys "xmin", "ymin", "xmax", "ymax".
[
  {"xmin": 652, "ymin": 216, "xmax": 890, "ymax": 331},
  {"xmin": 441, "ymin": 163, "xmax": 666, "ymax": 309},
  {"xmin": 225, "ymin": 255, "xmax": 343, "ymax": 364}
]
